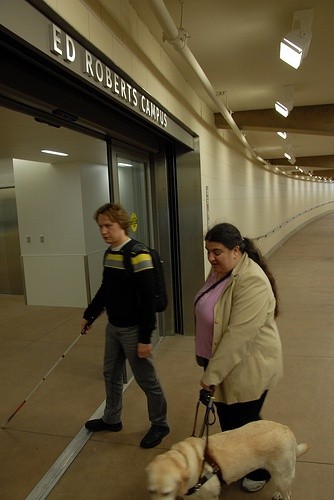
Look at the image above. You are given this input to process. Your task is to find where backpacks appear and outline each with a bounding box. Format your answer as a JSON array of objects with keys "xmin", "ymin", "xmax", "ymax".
[{"xmin": 123, "ymin": 241, "xmax": 168, "ymax": 310}]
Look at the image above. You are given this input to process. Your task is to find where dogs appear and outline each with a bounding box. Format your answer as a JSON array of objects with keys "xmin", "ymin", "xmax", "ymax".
[{"xmin": 144, "ymin": 419, "xmax": 306, "ymax": 500}]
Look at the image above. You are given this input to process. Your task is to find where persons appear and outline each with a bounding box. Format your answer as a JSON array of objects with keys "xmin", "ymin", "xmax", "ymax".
[
  {"xmin": 192, "ymin": 223, "xmax": 281, "ymax": 492},
  {"xmin": 83, "ymin": 203, "xmax": 170, "ymax": 448}
]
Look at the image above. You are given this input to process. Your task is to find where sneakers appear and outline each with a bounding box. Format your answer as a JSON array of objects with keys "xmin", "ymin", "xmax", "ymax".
[{"xmin": 242, "ymin": 478, "xmax": 266, "ymax": 492}]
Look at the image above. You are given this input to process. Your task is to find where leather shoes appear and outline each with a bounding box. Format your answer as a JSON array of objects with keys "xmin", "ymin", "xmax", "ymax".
[
  {"xmin": 85, "ymin": 420, "xmax": 122, "ymax": 432},
  {"xmin": 140, "ymin": 426, "xmax": 170, "ymax": 448}
]
